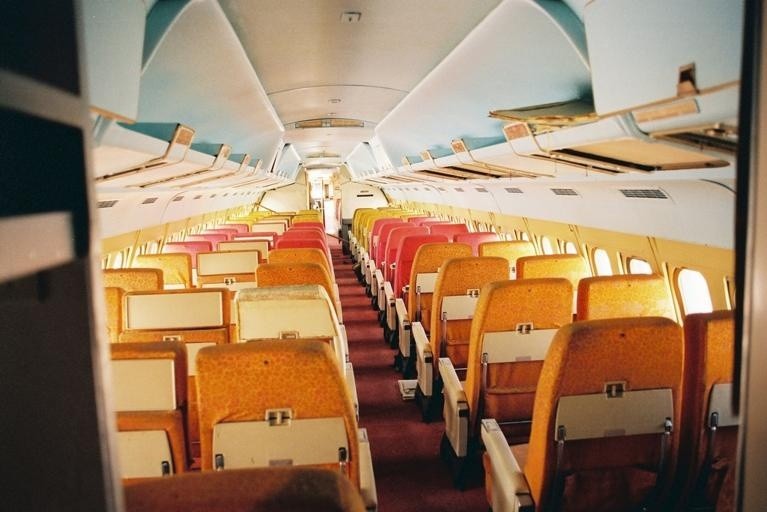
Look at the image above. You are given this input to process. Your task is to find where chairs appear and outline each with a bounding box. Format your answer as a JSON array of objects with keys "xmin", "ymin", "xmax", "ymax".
[{"xmin": 100, "ymin": 202, "xmax": 743, "ymax": 511}]
